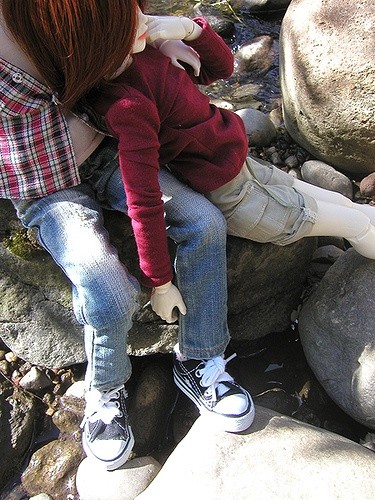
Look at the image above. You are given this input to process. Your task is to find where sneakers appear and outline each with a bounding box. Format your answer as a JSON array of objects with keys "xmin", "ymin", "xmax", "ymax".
[
  {"xmin": 172, "ymin": 344, "xmax": 255, "ymax": 433},
  {"xmin": 79, "ymin": 384, "xmax": 135, "ymax": 470}
]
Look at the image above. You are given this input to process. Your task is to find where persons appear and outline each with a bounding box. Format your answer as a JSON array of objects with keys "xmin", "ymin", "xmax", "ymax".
[
  {"xmin": 0, "ymin": 0, "xmax": 255, "ymax": 472},
  {"xmin": 0, "ymin": 0, "xmax": 375, "ymax": 323}
]
